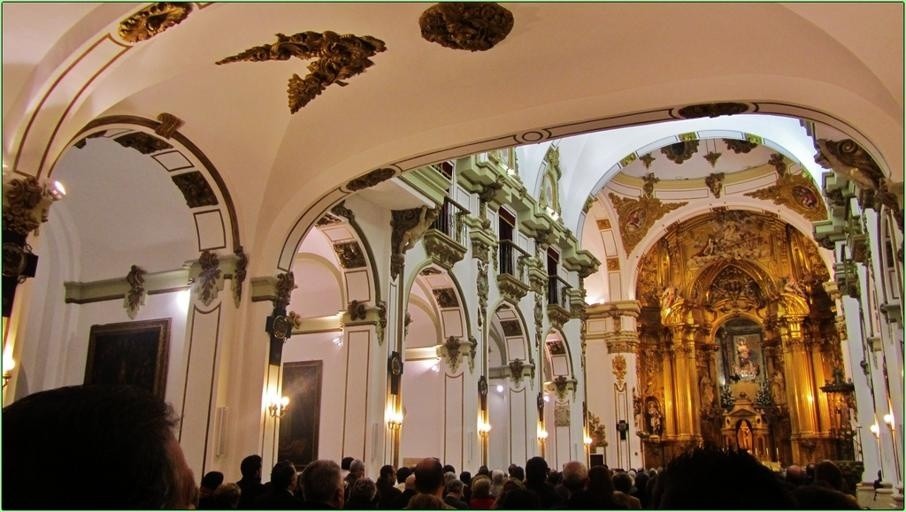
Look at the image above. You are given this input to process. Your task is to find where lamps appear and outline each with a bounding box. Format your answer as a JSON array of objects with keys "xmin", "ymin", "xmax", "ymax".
[
  {"xmin": 42, "ymin": 181, "xmax": 65, "ymax": 201},
  {"xmin": 2, "ymin": 346, "xmax": 17, "ymax": 386},
  {"xmin": 268, "ymin": 393, "xmax": 289, "ymax": 419}
]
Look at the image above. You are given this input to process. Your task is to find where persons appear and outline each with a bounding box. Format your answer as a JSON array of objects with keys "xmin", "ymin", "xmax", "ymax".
[
  {"xmin": 641, "ymin": 172, "xmax": 661, "ymax": 195},
  {"xmin": 199, "ymin": 440, "xmax": 863, "ymax": 511},
  {"xmin": 659, "ymin": 280, "xmax": 679, "ymax": 309},
  {"xmin": 872, "ymin": 469, "xmax": 885, "ymax": 502},
  {"xmin": 2, "ymin": 381, "xmax": 201, "ymax": 510},
  {"xmin": 707, "ymin": 172, "xmax": 723, "ymax": 196},
  {"xmin": 767, "ymin": 153, "xmax": 788, "ymax": 177},
  {"xmin": 736, "ymin": 339, "xmax": 753, "ymax": 367},
  {"xmin": 783, "ymin": 272, "xmax": 805, "ymax": 296}
]
